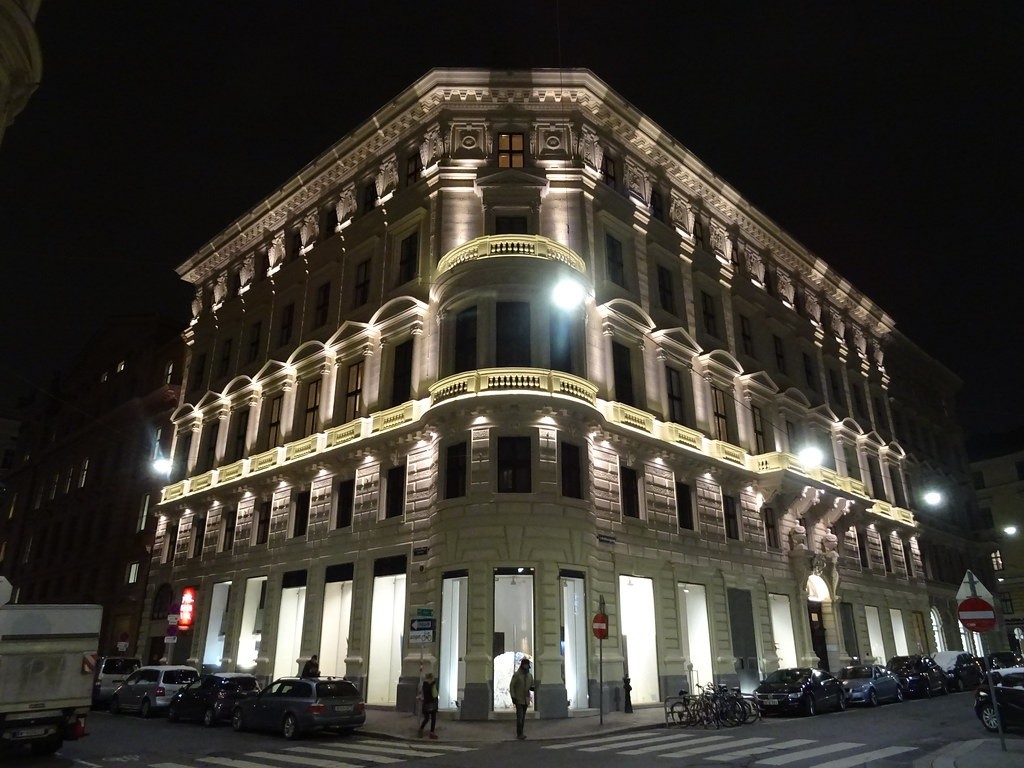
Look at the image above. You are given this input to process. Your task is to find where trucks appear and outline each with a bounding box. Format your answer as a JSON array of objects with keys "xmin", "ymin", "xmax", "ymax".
[{"xmin": 0, "ymin": 604, "xmax": 105, "ymax": 758}]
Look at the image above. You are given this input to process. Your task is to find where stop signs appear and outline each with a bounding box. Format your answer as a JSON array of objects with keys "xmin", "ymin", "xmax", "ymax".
[
  {"xmin": 958, "ymin": 598, "xmax": 996, "ymax": 631},
  {"xmin": 593, "ymin": 613, "xmax": 609, "ymax": 639}
]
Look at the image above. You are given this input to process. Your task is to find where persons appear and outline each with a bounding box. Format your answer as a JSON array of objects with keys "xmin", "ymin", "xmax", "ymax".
[
  {"xmin": 301, "ymin": 655, "xmax": 321, "ymax": 677},
  {"xmin": 417, "ymin": 672, "xmax": 440, "ymax": 739},
  {"xmin": 509, "ymin": 658, "xmax": 537, "ymax": 740}
]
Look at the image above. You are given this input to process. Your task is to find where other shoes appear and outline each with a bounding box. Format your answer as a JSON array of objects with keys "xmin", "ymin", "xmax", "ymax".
[
  {"xmin": 429, "ymin": 733, "xmax": 437, "ymax": 739},
  {"xmin": 418, "ymin": 728, "xmax": 423, "ymax": 738},
  {"xmin": 517, "ymin": 734, "xmax": 527, "ymax": 740}
]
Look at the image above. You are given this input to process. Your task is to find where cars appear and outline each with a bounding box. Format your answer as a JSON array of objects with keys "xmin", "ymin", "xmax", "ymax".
[
  {"xmin": 973, "ymin": 667, "xmax": 1024, "ymax": 732},
  {"xmin": 108, "ymin": 665, "xmax": 201, "ymax": 719},
  {"xmin": 986, "ymin": 652, "xmax": 1021, "ymax": 673},
  {"xmin": 833, "ymin": 664, "xmax": 905, "ymax": 707},
  {"xmin": 166, "ymin": 671, "xmax": 261, "ymax": 727},
  {"xmin": 752, "ymin": 667, "xmax": 845, "ymax": 717}
]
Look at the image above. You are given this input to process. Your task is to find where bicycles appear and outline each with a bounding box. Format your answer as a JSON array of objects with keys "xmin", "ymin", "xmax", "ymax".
[{"xmin": 672, "ymin": 684, "xmax": 762, "ymax": 729}]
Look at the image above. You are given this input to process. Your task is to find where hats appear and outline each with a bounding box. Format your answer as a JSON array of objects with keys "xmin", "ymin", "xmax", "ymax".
[{"xmin": 521, "ymin": 659, "xmax": 530, "ymax": 665}]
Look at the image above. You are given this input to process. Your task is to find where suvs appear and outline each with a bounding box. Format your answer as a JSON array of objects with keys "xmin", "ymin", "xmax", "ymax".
[
  {"xmin": 230, "ymin": 676, "xmax": 367, "ymax": 740},
  {"xmin": 929, "ymin": 651, "xmax": 984, "ymax": 693},
  {"xmin": 884, "ymin": 654, "xmax": 947, "ymax": 698},
  {"xmin": 92, "ymin": 656, "xmax": 142, "ymax": 702}
]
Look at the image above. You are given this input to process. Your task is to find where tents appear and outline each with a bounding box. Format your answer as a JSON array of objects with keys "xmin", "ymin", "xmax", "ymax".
[{"xmin": 493, "ymin": 652, "xmax": 534, "ymax": 708}]
